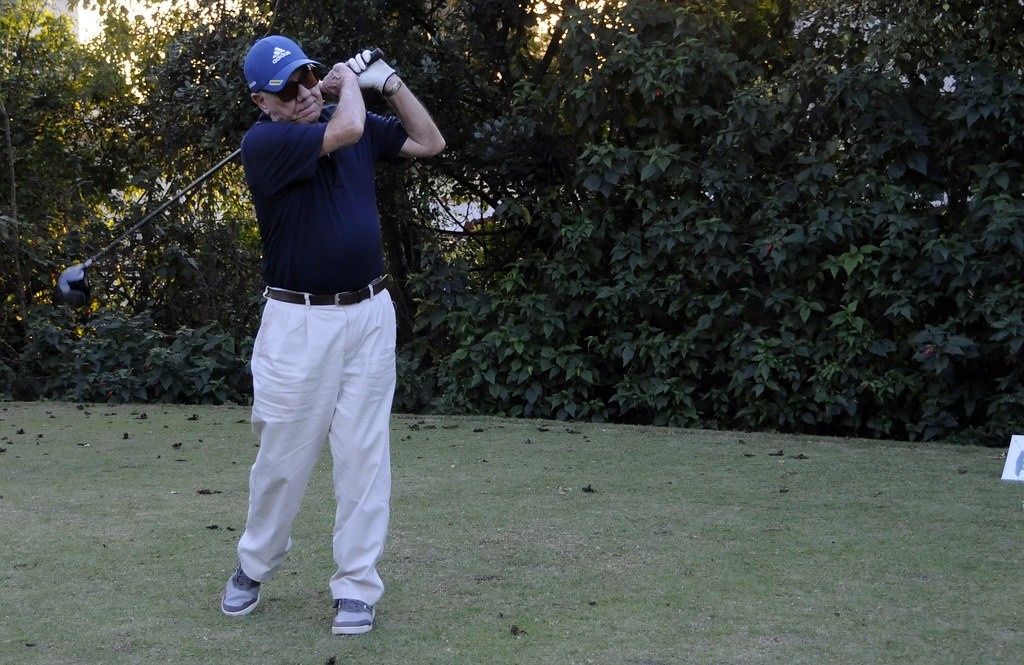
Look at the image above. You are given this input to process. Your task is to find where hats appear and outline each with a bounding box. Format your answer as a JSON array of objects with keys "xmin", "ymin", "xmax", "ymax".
[{"xmin": 243, "ymin": 35, "xmax": 322, "ymax": 93}]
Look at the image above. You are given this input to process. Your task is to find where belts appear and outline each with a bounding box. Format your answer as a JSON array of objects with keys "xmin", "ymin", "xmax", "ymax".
[{"xmin": 270, "ymin": 274, "xmax": 390, "ymax": 306}]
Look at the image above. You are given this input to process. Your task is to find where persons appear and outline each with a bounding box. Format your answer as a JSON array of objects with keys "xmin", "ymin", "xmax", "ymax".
[{"xmin": 220, "ymin": 34, "xmax": 446, "ymax": 635}]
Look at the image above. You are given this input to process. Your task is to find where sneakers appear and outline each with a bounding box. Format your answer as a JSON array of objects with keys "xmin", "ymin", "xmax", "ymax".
[
  {"xmin": 331, "ymin": 598, "xmax": 376, "ymax": 635},
  {"xmin": 221, "ymin": 563, "xmax": 261, "ymax": 616}
]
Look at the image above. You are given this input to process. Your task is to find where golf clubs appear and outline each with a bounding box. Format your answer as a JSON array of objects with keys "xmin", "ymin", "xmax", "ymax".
[{"xmin": 53, "ymin": 48, "xmax": 386, "ymax": 310}]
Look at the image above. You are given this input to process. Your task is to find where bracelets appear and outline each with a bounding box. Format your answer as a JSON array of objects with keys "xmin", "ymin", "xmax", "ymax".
[{"xmin": 384, "ymin": 78, "xmax": 403, "ymax": 97}]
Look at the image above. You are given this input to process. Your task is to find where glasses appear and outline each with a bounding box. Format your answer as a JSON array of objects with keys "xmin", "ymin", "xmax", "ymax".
[{"xmin": 260, "ymin": 68, "xmax": 320, "ymax": 103}]
